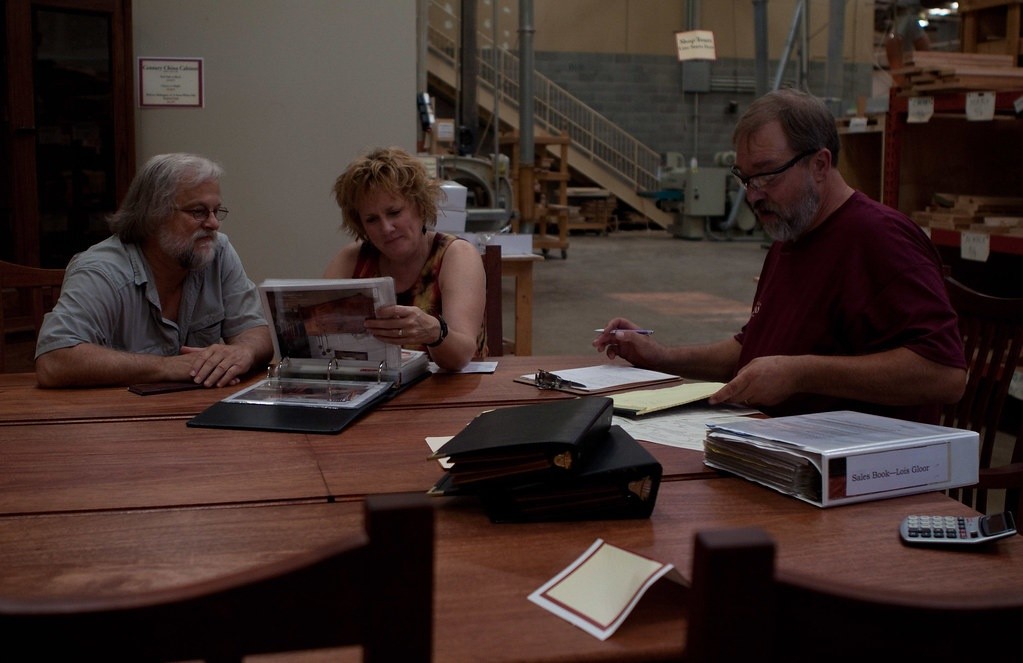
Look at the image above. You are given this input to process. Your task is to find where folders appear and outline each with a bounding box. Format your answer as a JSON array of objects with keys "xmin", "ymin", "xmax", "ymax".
[
  {"xmin": 423, "ymin": 396, "xmax": 614, "ymax": 499},
  {"xmin": 701, "ymin": 411, "xmax": 981, "ymax": 511},
  {"xmin": 185, "ymin": 276, "xmax": 432, "ymax": 435},
  {"xmin": 487, "ymin": 425, "xmax": 663, "ymax": 524}
]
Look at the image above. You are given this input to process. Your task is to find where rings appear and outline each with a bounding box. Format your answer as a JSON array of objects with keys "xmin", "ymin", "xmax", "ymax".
[{"xmin": 399, "ymin": 330, "xmax": 402, "ymax": 336}]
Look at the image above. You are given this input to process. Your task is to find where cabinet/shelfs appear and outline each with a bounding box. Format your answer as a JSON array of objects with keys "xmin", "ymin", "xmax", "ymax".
[{"xmin": 875, "ymin": 89, "xmax": 1023, "ymax": 256}]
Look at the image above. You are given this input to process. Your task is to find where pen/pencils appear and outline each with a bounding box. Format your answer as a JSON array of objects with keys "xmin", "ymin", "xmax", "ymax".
[{"xmin": 594, "ymin": 329, "xmax": 654, "ymax": 336}]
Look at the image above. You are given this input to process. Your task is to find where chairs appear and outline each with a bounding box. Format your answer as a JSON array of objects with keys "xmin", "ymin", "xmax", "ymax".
[{"xmin": 938, "ymin": 273, "xmax": 1022, "ymax": 532}]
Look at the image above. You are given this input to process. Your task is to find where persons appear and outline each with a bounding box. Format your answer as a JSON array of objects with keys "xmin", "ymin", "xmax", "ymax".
[
  {"xmin": 320, "ymin": 147, "xmax": 489, "ymax": 372},
  {"xmin": 34, "ymin": 153, "xmax": 274, "ymax": 390},
  {"xmin": 593, "ymin": 88, "xmax": 969, "ymax": 425}
]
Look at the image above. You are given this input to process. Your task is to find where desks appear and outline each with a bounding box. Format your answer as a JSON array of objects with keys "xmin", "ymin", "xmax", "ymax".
[{"xmin": 1, "ymin": 353, "xmax": 1023, "ymax": 662}]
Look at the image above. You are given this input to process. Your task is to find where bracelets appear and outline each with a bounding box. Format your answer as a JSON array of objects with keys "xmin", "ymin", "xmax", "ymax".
[{"xmin": 426, "ymin": 315, "xmax": 448, "ymax": 347}]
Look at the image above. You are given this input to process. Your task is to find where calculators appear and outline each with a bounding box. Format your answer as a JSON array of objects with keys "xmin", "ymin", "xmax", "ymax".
[{"xmin": 898, "ymin": 510, "xmax": 1017, "ymax": 549}]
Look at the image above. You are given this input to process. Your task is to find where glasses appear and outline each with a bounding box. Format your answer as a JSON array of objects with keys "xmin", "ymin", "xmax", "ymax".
[
  {"xmin": 170, "ymin": 204, "xmax": 230, "ymax": 222},
  {"xmin": 731, "ymin": 148, "xmax": 818, "ymax": 193}
]
